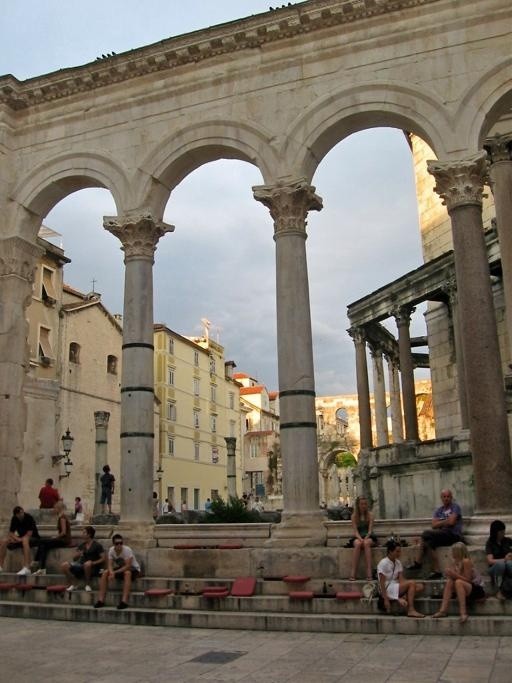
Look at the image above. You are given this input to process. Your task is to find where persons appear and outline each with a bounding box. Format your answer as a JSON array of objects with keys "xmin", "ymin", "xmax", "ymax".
[
  {"xmin": 349, "ymin": 488, "xmax": 512, "ymax": 623},
  {"xmin": 100, "ymin": 465, "xmax": 115, "ymax": 516},
  {"xmin": 0, "ymin": 478, "xmax": 142, "ymax": 609},
  {"xmin": 152, "ymin": 491, "xmax": 264, "ymax": 520}
]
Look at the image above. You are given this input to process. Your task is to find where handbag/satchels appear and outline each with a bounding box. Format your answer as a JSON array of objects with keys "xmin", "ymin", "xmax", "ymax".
[
  {"xmin": 363, "ymin": 582, "xmax": 378, "ymax": 598},
  {"xmin": 383, "ymin": 535, "xmax": 407, "ymax": 547}
]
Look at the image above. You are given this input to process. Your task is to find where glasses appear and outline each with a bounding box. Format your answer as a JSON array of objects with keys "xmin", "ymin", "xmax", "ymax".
[{"xmin": 115, "ymin": 542, "xmax": 122, "ymax": 545}]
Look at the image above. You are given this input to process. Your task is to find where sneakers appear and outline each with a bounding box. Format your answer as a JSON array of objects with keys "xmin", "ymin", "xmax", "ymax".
[
  {"xmin": 31, "ymin": 560, "xmax": 46, "ymax": 576},
  {"xmin": 405, "ymin": 561, "xmax": 422, "ymax": 570},
  {"xmin": 95, "ymin": 601, "xmax": 104, "ymax": 608},
  {"xmin": 67, "ymin": 585, "xmax": 78, "ymax": 591},
  {"xmin": 425, "ymin": 570, "xmax": 442, "ymax": 578},
  {"xmin": 85, "ymin": 584, "xmax": 92, "ymax": 591},
  {"xmin": 117, "ymin": 602, "xmax": 128, "ymax": 609},
  {"xmin": 17, "ymin": 568, "xmax": 31, "ymax": 576}
]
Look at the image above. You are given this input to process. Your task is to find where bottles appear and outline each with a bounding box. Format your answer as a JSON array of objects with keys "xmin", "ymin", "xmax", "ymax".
[
  {"xmin": 323, "ymin": 581, "xmax": 333, "ymax": 592},
  {"xmin": 99, "ymin": 569, "xmax": 104, "ymax": 576},
  {"xmin": 432, "ymin": 576, "xmax": 445, "ymax": 596},
  {"xmin": 255, "ymin": 567, "xmax": 266, "ymax": 584},
  {"xmin": 336, "ymin": 536, "xmax": 340, "ymax": 547}
]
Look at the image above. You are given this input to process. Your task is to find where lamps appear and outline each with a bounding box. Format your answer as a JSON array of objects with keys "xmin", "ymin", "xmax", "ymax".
[
  {"xmin": 52, "ymin": 426, "xmax": 74, "ymax": 467},
  {"xmin": 154, "ymin": 465, "xmax": 163, "ymax": 482},
  {"xmin": 59, "ymin": 458, "xmax": 73, "ymax": 481}
]
